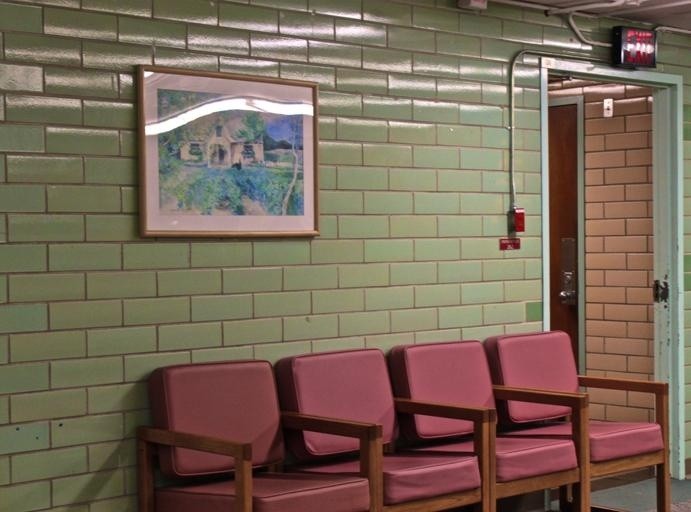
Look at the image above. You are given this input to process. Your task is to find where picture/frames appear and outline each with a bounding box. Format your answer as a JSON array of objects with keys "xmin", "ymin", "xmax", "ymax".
[{"xmin": 132, "ymin": 64, "xmax": 320, "ymax": 238}]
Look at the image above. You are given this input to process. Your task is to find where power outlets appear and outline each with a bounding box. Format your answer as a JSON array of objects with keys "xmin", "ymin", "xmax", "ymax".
[{"xmin": 603, "ymin": 98, "xmax": 613, "ymax": 117}]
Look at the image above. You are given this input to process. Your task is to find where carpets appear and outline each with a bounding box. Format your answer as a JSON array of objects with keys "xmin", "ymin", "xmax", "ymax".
[{"xmin": 649, "ymin": 499, "xmax": 691, "ymax": 512}]
